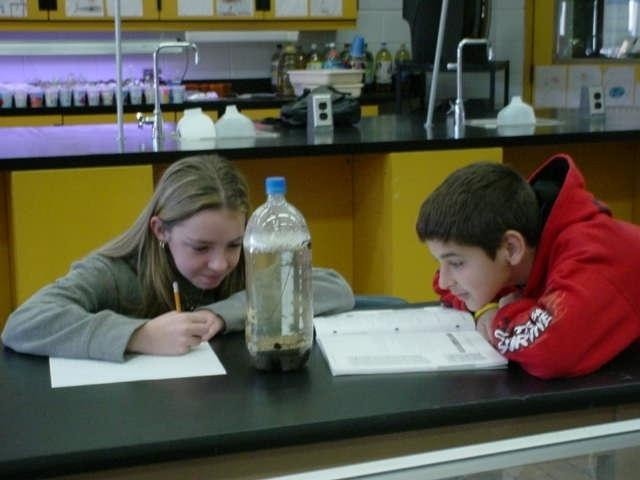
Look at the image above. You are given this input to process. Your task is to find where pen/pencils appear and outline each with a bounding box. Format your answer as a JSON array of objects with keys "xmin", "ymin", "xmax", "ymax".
[{"xmin": 172, "ymin": 282, "xmax": 180, "ymax": 311}]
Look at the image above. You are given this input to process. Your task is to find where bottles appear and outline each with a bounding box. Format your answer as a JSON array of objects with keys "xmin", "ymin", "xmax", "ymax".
[
  {"xmin": 242, "ymin": 176, "xmax": 314, "ymax": 373},
  {"xmin": 270, "ymin": 43, "xmax": 411, "ymax": 98}
]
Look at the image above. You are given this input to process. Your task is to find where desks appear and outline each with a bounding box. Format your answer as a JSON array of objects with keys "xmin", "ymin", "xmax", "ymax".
[
  {"xmin": 0, "ymin": 96, "xmax": 378, "ymax": 127},
  {"xmin": 1, "ymin": 107, "xmax": 640, "ymax": 349},
  {"xmin": 1, "ymin": 301, "xmax": 640, "ymax": 480}
]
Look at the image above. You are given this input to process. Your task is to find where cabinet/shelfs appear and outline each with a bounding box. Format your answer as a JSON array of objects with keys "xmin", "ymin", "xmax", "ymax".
[{"xmin": 0, "ymin": 0, "xmax": 360, "ymax": 32}]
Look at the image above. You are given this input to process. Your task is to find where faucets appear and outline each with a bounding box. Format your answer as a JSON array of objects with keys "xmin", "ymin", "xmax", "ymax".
[
  {"xmin": 457, "ymin": 39, "xmax": 493, "ymax": 100},
  {"xmin": 154, "ymin": 42, "xmax": 200, "ymax": 111}
]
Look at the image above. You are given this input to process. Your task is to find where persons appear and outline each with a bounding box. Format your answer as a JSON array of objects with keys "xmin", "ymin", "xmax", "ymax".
[
  {"xmin": 415, "ymin": 149, "xmax": 640, "ymax": 382},
  {"xmin": 0, "ymin": 153, "xmax": 357, "ymax": 364}
]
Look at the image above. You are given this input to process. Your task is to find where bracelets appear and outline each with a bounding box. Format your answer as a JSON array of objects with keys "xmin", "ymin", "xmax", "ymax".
[{"xmin": 472, "ymin": 302, "xmax": 499, "ymax": 321}]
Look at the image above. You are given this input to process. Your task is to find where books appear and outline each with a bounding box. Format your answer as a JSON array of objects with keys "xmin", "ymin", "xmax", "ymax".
[{"xmin": 306, "ymin": 304, "xmax": 512, "ymax": 381}]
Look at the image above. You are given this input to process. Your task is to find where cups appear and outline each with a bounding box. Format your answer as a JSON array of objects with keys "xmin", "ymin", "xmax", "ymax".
[{"xmin": 2, "ymin": 84, "xmax": 186, "ymax": 109}]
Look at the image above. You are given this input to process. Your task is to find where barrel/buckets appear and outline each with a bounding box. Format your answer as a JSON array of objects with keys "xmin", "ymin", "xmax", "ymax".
[
  {"xmin": 215, "ymin": 104, "xmax": 255, "ymax": 137},
  {"xmin": 498, "ymin": 96, "xmax": 535, "ymax": 126},
  {"xmin": 176, "ymin": 108, "xmax": 215, "ymax": 137}
]
